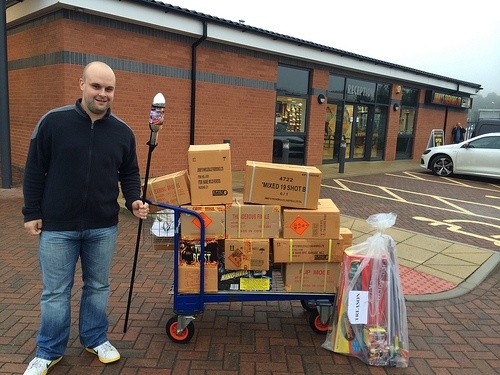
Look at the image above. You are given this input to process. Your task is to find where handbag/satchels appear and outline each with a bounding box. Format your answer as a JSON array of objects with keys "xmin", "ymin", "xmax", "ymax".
[{"xmin": 321, "ymin": 211, "xmax": 410, "ymax": 369}]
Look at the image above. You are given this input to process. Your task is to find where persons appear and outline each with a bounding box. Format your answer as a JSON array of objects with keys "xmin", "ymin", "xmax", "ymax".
[
  {"xmin": 22, "ymin": 61, "xmax": 150, "ymax": 375},
  {"xmin": 451, "ymin": 123, "xmax": 466, "ymax": 144}
]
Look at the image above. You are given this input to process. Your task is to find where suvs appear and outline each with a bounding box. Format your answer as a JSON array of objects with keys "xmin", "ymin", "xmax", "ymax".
[{"xmin": 471, "ymin": 120, "xmax": 500, "ymax": 137}]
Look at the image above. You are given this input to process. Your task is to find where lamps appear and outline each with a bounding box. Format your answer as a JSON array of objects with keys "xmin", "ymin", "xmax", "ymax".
[
  {"xmin": 393, "ymin": 102, "xmax": 400, "ymax": 112},
  {"xmin": 318, "ymin": 94, "xmax": 325, "ymax": 104}
]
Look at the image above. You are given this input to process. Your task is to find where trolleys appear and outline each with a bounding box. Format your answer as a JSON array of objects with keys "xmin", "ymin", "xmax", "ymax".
[{"xmin": 138, "ymin": 192, "xmax": 338, "ymax": 344}]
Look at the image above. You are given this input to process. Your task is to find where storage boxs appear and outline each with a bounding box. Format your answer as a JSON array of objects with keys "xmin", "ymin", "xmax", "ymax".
[{"xmin": 140, "ymin": 143, "xmax": 390, "ymax": 366}]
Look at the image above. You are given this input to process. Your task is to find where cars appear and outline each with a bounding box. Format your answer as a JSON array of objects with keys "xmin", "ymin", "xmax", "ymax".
[
  {"xmin": 420, "ymin": 133, "xmax": 500, "ymax": 180},
  {"xmin": 273, "ymin": 130, "xmax": 305, "ymax": 158}
]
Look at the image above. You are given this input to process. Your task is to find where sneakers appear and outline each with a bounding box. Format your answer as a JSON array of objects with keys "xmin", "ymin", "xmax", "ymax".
[
  {"xmin": 85, "ymin": 340, "xmax": 121, "ymax": 363},
  {"xmin": 22, "ymin": 355, "xmax": 62, "ymax": 375}
]
